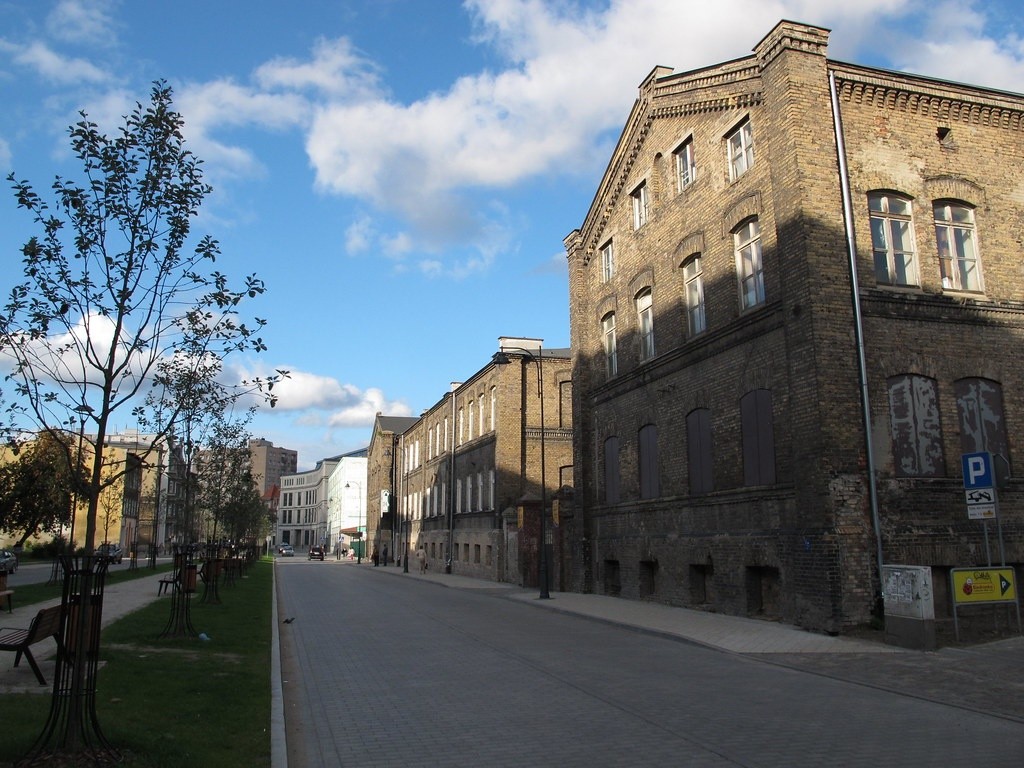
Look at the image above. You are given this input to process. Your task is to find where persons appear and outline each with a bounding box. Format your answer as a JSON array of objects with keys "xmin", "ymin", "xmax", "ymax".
[
  {"xmin": 373, "ymin": 545, "xmax": 379, "ymax": 567},
  {"xmin": 382, "ymin": 544, "xmax": 388, "ymax": 566},
  {"xmin": 416, "ymin": 546, "xmax": 426, "ymax": 575}
]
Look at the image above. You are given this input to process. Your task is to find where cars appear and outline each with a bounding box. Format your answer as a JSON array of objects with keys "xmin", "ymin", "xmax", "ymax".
[
  {"xmin": 0, "ymin": 549, "xmax": 19, "ymax": 574},
  {"xmin": 283, "ymin": 546, "xmax": 294, "ymax": 557},
  {"xmin": 279, "ymin": 544, "xmax": 288, "ymax": 553},
  {"xmin": 93, "ymin": 544, "xmax": 123, "ymax": 564},
  {"xmin": 188, "ymin": 543, "xmax": 200, "ymax": 552},
  {"xmin": 308, "ymin": 546, "xmax": 325, "ymax": 561}
]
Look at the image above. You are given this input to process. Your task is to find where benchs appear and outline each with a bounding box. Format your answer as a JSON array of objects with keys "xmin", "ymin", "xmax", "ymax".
[
  {"xmin": 0, "ymin": 600, "xmax": 75, "ymax": 684},
  {"xmin": 158, "ymin": 570, "xmax": 181, "ymax": 596},
  {"xmin": 0, "ymin": 576, "xmax": 15, "ymax": 613},
  {"xmin": 196, "ymin": 565, "xmax": 205, "ymax": 581}
]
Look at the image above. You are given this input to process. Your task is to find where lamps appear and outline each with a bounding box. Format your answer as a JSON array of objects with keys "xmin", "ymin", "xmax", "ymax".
[{"xmin": 936, "ymin": 127, "xmax": 951, "ymax": 144}]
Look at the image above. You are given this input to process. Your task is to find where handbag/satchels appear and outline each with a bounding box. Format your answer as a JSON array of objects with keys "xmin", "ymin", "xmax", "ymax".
[{"xmin": 417, "ymin": 553, "xmax": 419, "ymax": 556}]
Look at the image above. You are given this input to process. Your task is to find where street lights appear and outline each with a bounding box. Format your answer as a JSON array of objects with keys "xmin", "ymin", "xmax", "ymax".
[
  {"xmin": 154, "ymin": 464, "xmax": 167, "ymax": 567},
  {"xmin": 329, "ymin": 496, "xmax": 341, "ymax": 560},
  {"xmin": 382, "ymin": 446, "xmax": 408, "ymax": 572},
  {"xmin": 70, "ymin": 405, "xmax": 96, "ymax": 545},
  {"xmin": 345, "ymin": 480, "xmax": 361, "ymax": 564},
  {"xmin": 493, "ymin": 346, "xmax": 549, "ymax": 598}
]
